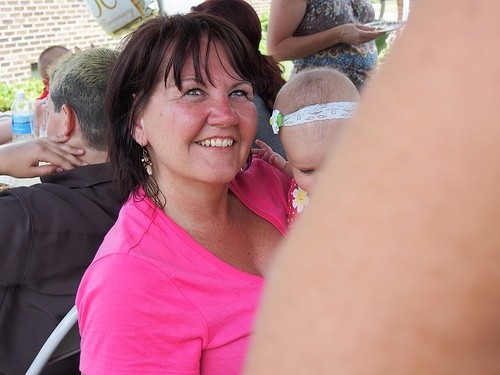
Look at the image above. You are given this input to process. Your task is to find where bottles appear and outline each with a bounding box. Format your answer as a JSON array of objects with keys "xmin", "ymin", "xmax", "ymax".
[{"xmin": 12, "ymin": 89, "xmax": 39, "ymax": 167}]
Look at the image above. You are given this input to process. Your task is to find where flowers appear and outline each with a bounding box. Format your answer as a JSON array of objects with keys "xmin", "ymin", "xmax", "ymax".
[{"xmin": 270, "ymin": 109, "xmax": 282, "ymax": 135}]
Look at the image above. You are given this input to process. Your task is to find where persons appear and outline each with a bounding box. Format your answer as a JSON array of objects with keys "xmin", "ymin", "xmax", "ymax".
[
  {"xmin": 75, "ymin": 12, "xmax": 291, "ymax": 375},
  {"xmin": 1, "ymin": 46, "xmax": 73, "ymax": 145},
  {"xmin": 266, "ymin": 0, "xmax": 378, "ymax": 89},
  {"xmin": 242, "ymin": 0, "xmax": 500, "ymax": 375},
  {"xmin": 250, "ymin": 67, "xmax": 360, "ymax": 193},
  {"xmin": 191, "ymin": 0, "xmax": 262, "ymax": 50},
  {"xmin": 0, "ymin": 48, "xmax": 120, "ymax": 375}
]
